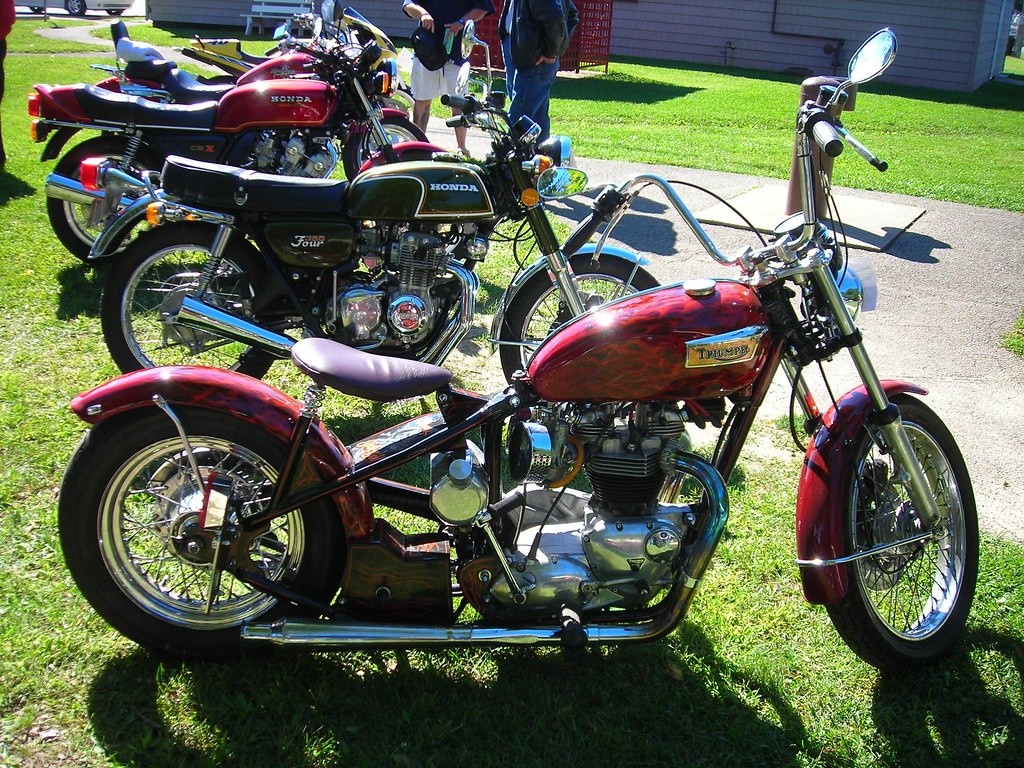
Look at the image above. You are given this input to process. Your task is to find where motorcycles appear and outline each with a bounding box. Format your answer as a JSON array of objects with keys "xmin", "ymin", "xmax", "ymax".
[{"xmin": 25, "ymin": 6, "xmax": 983, "ymax": 672}]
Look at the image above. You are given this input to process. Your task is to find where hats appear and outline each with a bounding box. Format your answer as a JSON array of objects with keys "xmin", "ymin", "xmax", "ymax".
[{"xmin": 414, "ymin": 19, "xmax": 446, "ymax": 63}]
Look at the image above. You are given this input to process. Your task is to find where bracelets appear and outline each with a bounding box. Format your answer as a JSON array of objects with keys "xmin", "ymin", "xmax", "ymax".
[
  {"xmin": 458, "ymin": 18, "xmax": 466, "ymax": 26},
  {"xmin": 420, "ymin": 13, "xmax": 429, "ymax": 20}
]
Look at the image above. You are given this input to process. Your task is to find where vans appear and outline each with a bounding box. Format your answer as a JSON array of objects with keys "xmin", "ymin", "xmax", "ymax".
[{"xmin": 13, "ymin": 0, "xmax": 136, "ymax": 15}]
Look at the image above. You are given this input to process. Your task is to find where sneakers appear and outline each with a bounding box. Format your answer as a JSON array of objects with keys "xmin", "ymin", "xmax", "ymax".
[{"xmin": 457, "ymin": 149, "xmax": 470, "ymax": 158}]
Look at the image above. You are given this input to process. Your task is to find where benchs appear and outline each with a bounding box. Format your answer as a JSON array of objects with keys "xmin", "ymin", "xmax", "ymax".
[{"xmin": 239, "ymin": 0, "xmax": 313, "ymax": 37}]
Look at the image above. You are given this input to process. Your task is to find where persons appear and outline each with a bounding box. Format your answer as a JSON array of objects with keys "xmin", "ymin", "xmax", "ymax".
[
  {"xmin": 0, "ymin": 0, "xmax": 16, "ymax": 173},
  {"xmin": 498, "ymin": 0, "xmax": 579, "ymax": 148},
  {"xmin": 402, "ymin": 0, "xmax": 497, "ymax": 155}
]
